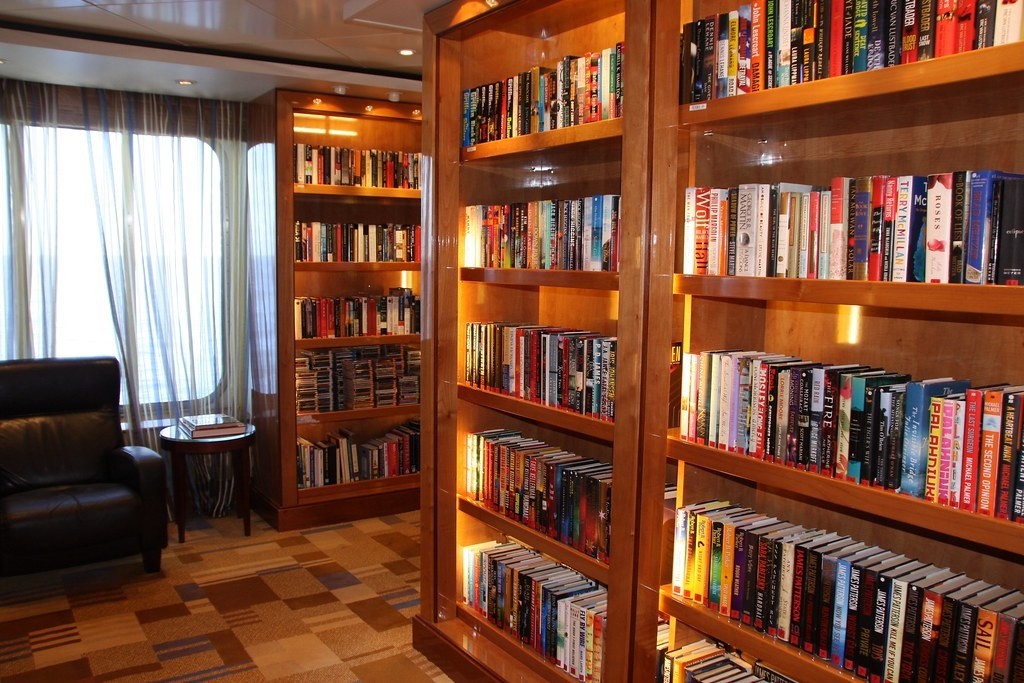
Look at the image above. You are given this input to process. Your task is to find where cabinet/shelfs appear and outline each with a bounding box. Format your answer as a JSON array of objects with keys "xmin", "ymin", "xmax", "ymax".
[
  {"xmin": 243, "ymin": 85, "xmax": 420, "ymax": 533},
  {"xmin": 632, "ymin": 0, "xmax": 1024, "ymax": 683},
  {"xmin": 413, "ymin": 0, "xmax": 653, "ymax": 683}
]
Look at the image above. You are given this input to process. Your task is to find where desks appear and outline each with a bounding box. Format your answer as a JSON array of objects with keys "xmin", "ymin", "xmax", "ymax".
[{"xmin": 159, "ymin": 424, "xmax": 256, "ymax": 543}]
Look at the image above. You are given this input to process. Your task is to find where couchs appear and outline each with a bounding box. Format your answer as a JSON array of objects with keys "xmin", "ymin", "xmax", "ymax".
[{"xmin": 0, "ymin": 356, "xmax": 168, "ymax": 573}]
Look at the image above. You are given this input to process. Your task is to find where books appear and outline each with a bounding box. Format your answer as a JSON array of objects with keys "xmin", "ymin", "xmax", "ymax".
[
  {"xmin": 180, "ymin": 411, "xmax": 245, "ymax": 438},
  {"xmin": 293, "ymin": 139, "xmax": 422, "ymax": 489},
  {"xmin": 461, "ymin": 42, "xmax": 624, "ymax": 683},
  {"xmin": 656, "ymin": 2, "xmax": 1024, "ymax": 682}
]
